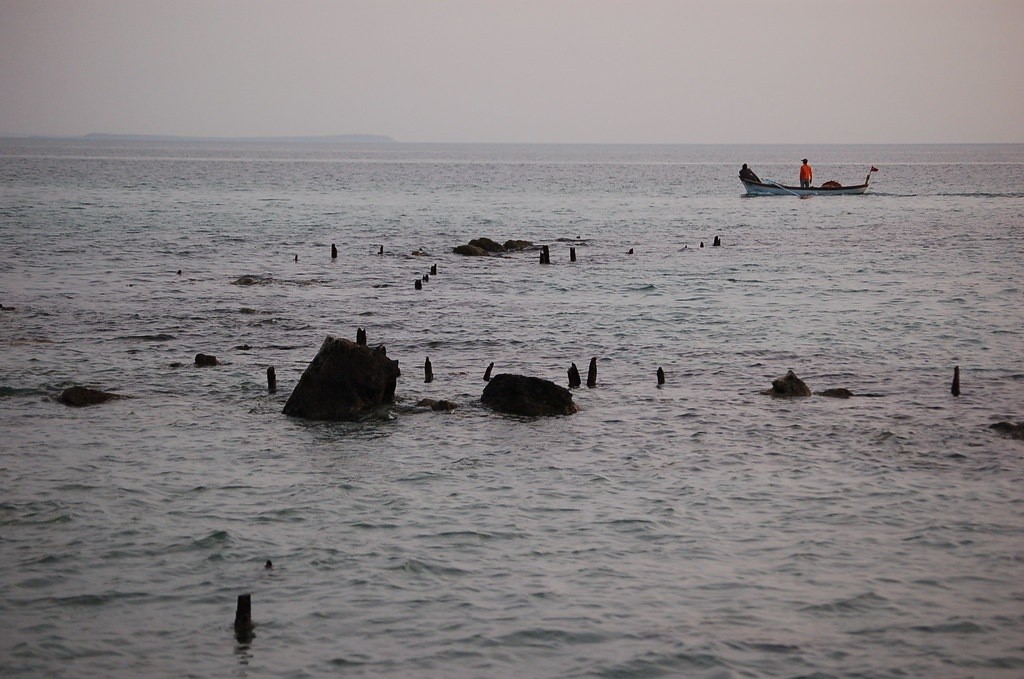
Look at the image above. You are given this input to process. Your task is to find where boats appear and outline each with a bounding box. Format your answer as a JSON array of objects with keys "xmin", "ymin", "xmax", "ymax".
[{"xmin": 737, "ymin": 163, "xmax": 869, "ymax": 196}]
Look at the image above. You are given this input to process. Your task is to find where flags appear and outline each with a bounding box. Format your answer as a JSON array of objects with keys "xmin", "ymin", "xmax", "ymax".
[{"xmin": 871, "ymin": 166, "xmax": 879, "ymax": 172}]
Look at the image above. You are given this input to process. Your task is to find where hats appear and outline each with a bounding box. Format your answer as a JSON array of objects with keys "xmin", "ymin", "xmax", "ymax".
[{"xmin": 801, "ymin": 159, "xmax": 809, "ymax": 161}]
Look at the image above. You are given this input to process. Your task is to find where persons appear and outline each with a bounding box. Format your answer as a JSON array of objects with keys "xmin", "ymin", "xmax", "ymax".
[
  {"xmin": 799, "ymin": 159, "xmax": 813, "ymax": 189},
  {"xmin": 739, "ymin": 163, "xmax": 763, "ymax": 184}
]
